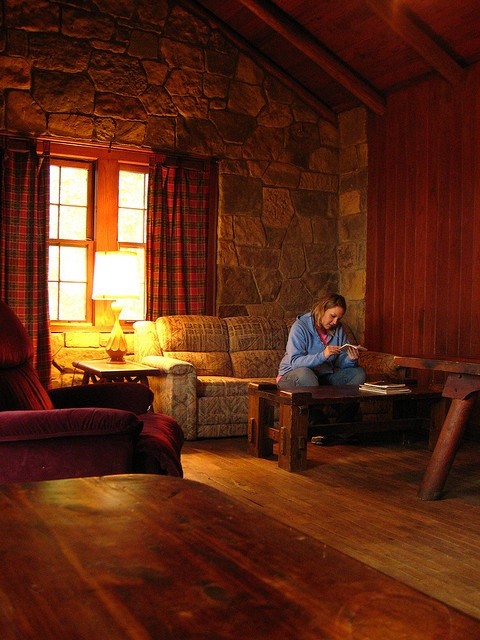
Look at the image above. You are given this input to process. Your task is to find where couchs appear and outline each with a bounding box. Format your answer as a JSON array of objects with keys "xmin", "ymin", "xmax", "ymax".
[
  {"xmin": 0, "ymin": 299, "xmax": 185, "ymax": 479},
  {"xmin": 133, "ymin": 312, "xmax": 410, "ymax": 442}
]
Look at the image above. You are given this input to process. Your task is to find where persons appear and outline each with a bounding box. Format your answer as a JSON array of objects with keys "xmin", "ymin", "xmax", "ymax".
[{"xmin": 276, "ymin": 293, "xmax": 366, "ymax": 446}]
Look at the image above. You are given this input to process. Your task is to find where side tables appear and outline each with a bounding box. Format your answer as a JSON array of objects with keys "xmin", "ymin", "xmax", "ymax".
[{"xmin": 71, "ymin": 357, "xmax": 161, "ymax": 392}]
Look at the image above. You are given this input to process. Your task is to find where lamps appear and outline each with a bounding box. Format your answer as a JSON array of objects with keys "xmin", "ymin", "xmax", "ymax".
[{"xmin": 90, "ymin": 251, "xmax": 145, "ymax": 366}]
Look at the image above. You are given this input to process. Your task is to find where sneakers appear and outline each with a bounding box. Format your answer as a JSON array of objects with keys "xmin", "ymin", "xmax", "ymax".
[{"xmin": 312, "ymin": 436, "xmax": 325, "ymax": 445}]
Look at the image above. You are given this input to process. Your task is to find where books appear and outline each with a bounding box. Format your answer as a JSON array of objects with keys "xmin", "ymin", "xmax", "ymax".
[
  {"xmin": 359, "ymin": 381, "xmax": 412, "ymax": 395},
  {"xmin": 338, "ymin": 343, "xmax": 368, "ymax": 355}
]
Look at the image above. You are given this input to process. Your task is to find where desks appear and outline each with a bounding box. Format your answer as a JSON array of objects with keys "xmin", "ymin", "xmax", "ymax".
[
  {"xmin": 391, "ymin": 353, "xmax": 479, "ymax": 499},
  {"xmin": 8, "ymin": 472, "xmax": 480, "ymax": 640},
  {"xmin": 247, "ymin": 382, "xmax": 448, "ymax": 473}
]
